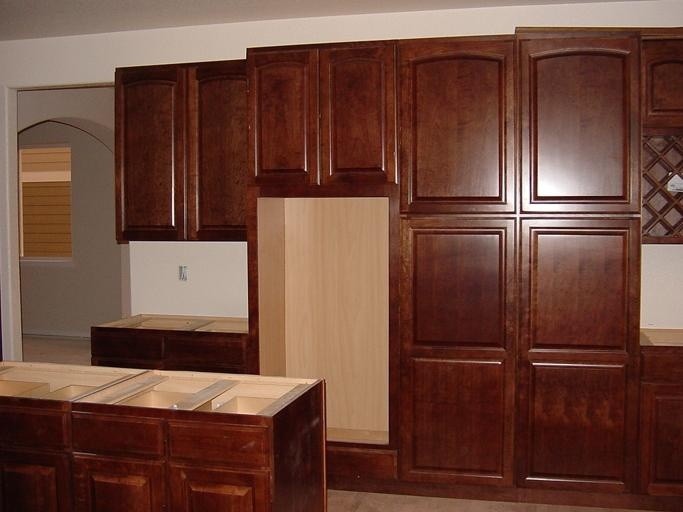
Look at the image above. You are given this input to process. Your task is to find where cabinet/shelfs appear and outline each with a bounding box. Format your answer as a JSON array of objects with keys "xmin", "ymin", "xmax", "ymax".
[
  {"xmin": 246, "ymin": 186, "xmax": 398, "ymax": 496},
  {"xmin": 640, "ymin": 28, "xmax": 683, "ymax": 244},
  {"xmin": 640, "ymin": 345, "xmax": 683, "ymax": 512},
  {"xmin": 167, "ymin": 381, "xmax": 326, "ymax": 512},
  {"xmin": 90, "ymin": 327, "xmax": 247, "ymax": 374},
  {"xmin": 70, "ymin": 401, "xmax": 166, "ymax": 512},
  {"xmin": 246, "ymin": 46, "xmax": 396, "ymax": 184},
  {"xmin": 114, "ymin": 60, "xmax": 246, "ymax": 244},
  {"xmin": 0, "ymin": 396, "xmax": 71, "ymax": 512},
  {"xmin": 398, "ymin": 25, "xmax": 640, "ymax": 508}
]
